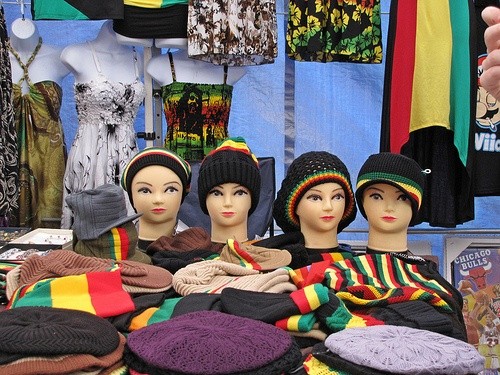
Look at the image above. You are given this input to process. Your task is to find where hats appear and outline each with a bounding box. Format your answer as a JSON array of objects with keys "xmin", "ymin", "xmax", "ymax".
[
  {"xmin": 65, "ymin": 184, "xmax": 143, "ymax": 241},
  {"xmin": 198, "ymin": 136, "xmax": 261, "ymax": 217},
  {"xmin": 355, "ymin": 153, "xmax": 426, "ymax": 227},
  {"xmin": 0, "ymin": 222, "xmax": 486, "ymax": 375},
  {"xmin": 121, "ymin": 147, "xmax": 192, "ymax": 213},
  {"xmin": 271, "ymin": 151, "xmax": 357, "ymax": 234}
]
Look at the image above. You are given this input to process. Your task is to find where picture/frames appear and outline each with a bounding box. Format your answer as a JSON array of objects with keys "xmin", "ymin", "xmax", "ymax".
[{"xmin": 443, "ymin": 235, "xmax": 500, "ymax": 372}]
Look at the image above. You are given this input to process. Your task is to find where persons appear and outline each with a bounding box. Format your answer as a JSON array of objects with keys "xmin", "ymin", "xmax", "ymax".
[
  {"xmin": 60, "ymin": 20, "xmax": 148, "ymax": 228},
  {"xmin": 146, "ymin": 47, "xmax": 247, "ymax": 165},
  {"xmin": 8, "ymin": 18, "xmax": 71, "ymax": 228},
  {"xmin": 480, "ymin": 6, "xmax": 500, "ymax": 102},
  {"xmin": 355, "ymin": 152, "xmax": 426, "ymax": 253},
  {"xmin": 121, "ymin": 147, "xmax": 192, "ymax": 241},
  {"xmin": 198, "ymin": 135, "xmax": 261, "ymax": 244},
  {"xmin": 272, "ymin": 151, "xmax": 358, "ymax": 250}
]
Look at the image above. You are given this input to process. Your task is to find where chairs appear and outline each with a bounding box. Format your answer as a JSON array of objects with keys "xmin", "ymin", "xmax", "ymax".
[{"xmin": 176, "ymin": 156, "xmax": 277, "ymax": 242}]
